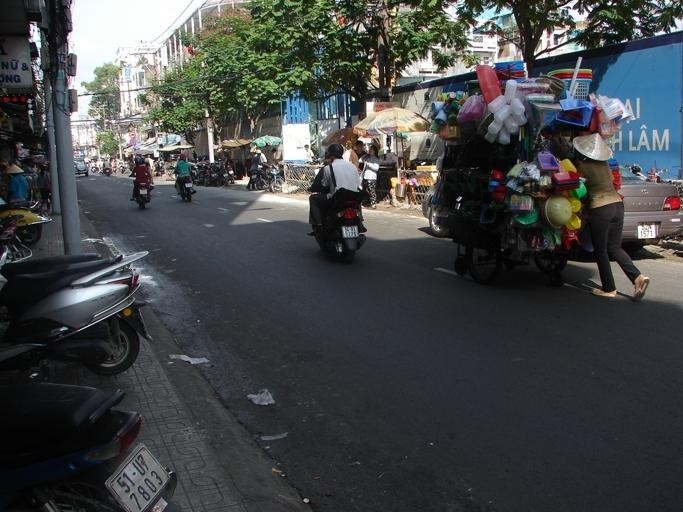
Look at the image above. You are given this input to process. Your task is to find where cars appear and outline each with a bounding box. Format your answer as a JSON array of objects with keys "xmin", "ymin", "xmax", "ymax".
[{"xmin": 419, "ymin": 157, "xmax": 682, "ymax": 261}]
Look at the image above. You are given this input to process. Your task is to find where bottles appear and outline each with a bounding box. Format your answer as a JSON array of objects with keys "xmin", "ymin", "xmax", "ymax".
[{"xmin": 484, "ymin": 80, "xmax": 529, "ymax": 149}]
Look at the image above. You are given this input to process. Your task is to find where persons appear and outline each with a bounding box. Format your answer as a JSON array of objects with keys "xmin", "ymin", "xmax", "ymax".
[
  {"xmin": 305, "ymin": 141, "xmax": 380, "ymax": 236},
  {"xmin": 0, "ymin": 161, "xmax": 51, "ymax": 211},
  {"xmin": 246, "ymin": 150, "xmax": 283, "ymax": 190},
  {"xmin": 89, "ymin": 154, "xmax": 196, "ymax": 203},
  {"xmin": 572, "ymin": 132, "xmax": 649, "ymax": 302}
]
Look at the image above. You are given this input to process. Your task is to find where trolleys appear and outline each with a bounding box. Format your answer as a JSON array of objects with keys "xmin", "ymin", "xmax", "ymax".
[{"xmin": 446, "ymin": 217, "xmax": 570, "ymax": 283}]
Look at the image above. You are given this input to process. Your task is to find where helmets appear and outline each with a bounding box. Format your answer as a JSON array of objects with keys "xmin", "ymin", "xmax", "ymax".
[
  {"xmin": 135, "ymin": 156, "xmax": 146, "ymax": 164},
  {"xmin": 328, "ymin": 144, "xmax": 343, "ymax": 159}
]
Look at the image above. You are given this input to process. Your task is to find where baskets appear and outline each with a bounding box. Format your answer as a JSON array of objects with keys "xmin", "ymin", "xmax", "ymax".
[{"xmin": 548, "ymin": 68, "xmax": 593, "ymax": 100}]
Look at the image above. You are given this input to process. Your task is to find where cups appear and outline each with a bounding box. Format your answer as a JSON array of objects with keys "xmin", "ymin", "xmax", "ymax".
[{"xmin": 430, "ymin": 92, "xmax": 460, "ymax": 125}]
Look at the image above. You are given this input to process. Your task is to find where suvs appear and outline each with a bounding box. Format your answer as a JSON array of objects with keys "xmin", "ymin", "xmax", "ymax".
[{"xmin": 74, "ymin": 158, "xmax": 88, "ymax": 177}]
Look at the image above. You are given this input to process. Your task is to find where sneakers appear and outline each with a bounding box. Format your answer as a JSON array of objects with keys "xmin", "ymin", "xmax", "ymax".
[
  {"xmin": 591, "ymin": 287, "xmax": 616, "ymax": 298},
  {"xmin": 307, "ymin": 227, "xmax": 323, "ymax": 236},
  {"xmin": 632, "ymin": 276, "xmax": 650, "ymax": 301}
]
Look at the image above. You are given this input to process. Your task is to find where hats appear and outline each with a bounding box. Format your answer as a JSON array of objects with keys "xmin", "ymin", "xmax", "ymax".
[
  {"xmin": 573, "ymin": 132, "xmax": 612, "ymax": 162},
  {"xmin": 6, "ymin": 163, "xmax": 25, "ymax": 174}
]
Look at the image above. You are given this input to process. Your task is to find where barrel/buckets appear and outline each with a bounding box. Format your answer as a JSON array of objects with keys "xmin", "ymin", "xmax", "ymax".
[
  {"xmin": 456, "ymin": 94, "xmax": 484, "ymax": 121},
  {"xmin": 485, "ymin": 80, "xmax": 528, "ymax": 145}
]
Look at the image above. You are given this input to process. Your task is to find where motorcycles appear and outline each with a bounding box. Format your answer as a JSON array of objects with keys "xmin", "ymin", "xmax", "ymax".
[
  {"xmin": 174, "ymin": 176, "xmax": 196, "ymax": 202},
  {"xmin": 128, "ymin": 173, "xmax": 154, "ymax": 208},
  {"xmin": 90, "ymin": 163, "xmax": 126, "ymax": 176},
  {"xmin": 247, "ymin": 162, "xmax": 284, "ymax": 192},
  {"xmin": 189, "ymin": 157, "xmax": 236, "ymax": 188},
  {"xmin": 305, "ymin": 182, "xmax": 367, "ymax": 265}
]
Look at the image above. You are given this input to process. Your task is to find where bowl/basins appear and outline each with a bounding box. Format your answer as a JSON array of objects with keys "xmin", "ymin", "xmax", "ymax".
[{"xmin": 513, "ymin": 178, "xmax": 589, "ymax": 231}]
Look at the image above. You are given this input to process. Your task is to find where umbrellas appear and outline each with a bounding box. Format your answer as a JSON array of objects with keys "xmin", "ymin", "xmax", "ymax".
[
  {"xmin": 322, "ymin": 128, "xmax": 361, "ymax": 146},
  {"xmin": 250, "ymin": 135, "xmax": 282, "ymax": 148},
  {"xmin": 352, "ymin": 107, "xmax": 431, "ymax": 156}
]
